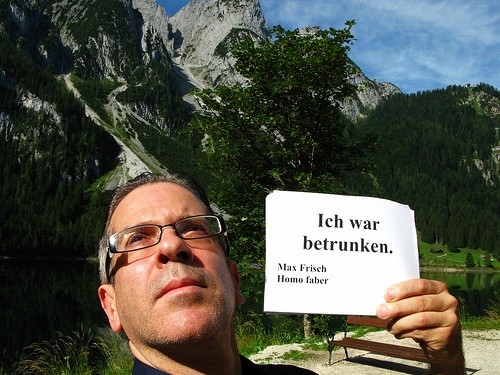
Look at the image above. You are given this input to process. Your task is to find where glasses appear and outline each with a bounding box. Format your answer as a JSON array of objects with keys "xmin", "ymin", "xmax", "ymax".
[{"xmin": 105, "ymin": 215, "xmax": 230, "ymax": 282}]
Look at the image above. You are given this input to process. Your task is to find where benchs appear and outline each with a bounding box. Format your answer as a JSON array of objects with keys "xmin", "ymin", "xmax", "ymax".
[{"xmin": 326, "ymin": 315, "xmax": 429, "ymax": 366}]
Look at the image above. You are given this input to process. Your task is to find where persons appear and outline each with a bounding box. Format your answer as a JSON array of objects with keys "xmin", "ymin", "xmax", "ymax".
[{"xmin": 96, "ymin": 172, "xmax": 467, "ymax": 375}]
